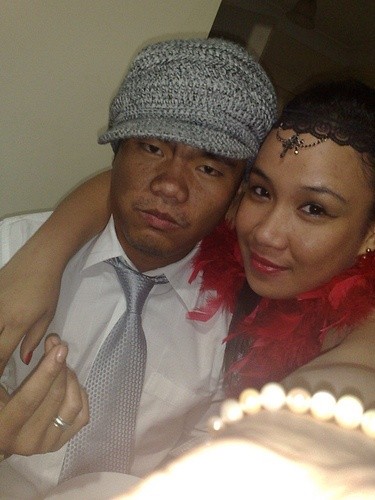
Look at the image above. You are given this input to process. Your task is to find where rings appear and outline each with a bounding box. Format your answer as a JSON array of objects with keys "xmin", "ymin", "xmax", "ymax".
[{"xmin": 53, "ymin": 415, "xmax": 70, "ymax": 430}]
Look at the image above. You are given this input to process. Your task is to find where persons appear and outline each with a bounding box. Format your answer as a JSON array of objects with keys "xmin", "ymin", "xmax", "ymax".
[
  {"xmin": 0, "ymin": 37, "xmax": 278, "ymax": 497},
  {"xmin": 0, "ymin": 72, "xmax": 375, "ymax": 500}
]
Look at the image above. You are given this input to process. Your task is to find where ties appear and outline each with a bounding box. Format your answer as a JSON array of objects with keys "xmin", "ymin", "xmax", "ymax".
[{"xmin": 58, "ymin": 258, "xmax": 170, "ymax": 488}]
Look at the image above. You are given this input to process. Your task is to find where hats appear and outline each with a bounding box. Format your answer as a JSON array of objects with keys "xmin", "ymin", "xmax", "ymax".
[{"xmin": 96, "ymin": 36, "xmax": 283, "ymax": 165}]
